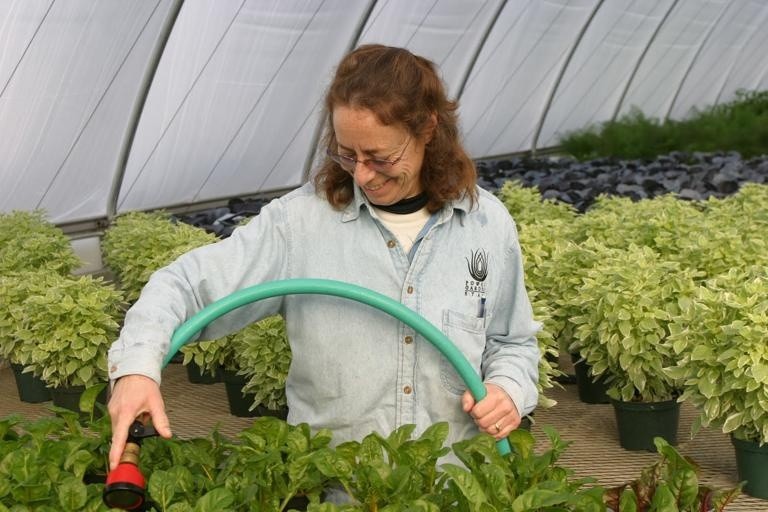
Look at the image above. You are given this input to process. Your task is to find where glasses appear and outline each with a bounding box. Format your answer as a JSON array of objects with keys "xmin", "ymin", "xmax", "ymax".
[{"xmin": 325, "ymin": 132, "xmax": 415, "ymax": 173}]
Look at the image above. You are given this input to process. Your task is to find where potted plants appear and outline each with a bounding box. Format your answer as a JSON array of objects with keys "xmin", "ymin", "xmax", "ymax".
[
  {"xmin": 672, "ymin": 269, "xmax": 767, "ymax": 501},
  {"xmin": 576, "ymin": 247, "xmax": 684, "ymax": 451},
  {"xmin": 49, "ymin": 273, "xmax": 128, "ymax": 420},
  {"xmin": 4, "ymin": 272, "xmax": 56, "ymax": 405},
  {"xmin": 183, "ymin": 337, "xmax": 225, "ymax": 384},
  {"xmin": 552, "ymin": 242, "xmax": 615, "ymax": 406},
  {"xmin": 226, "ymin": 313, "xmax": 286, "ymax": 418}
]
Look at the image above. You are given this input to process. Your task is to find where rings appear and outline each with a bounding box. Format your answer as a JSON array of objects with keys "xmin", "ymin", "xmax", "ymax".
[{"xmin": 495, "ymin": 423, "xmax": 500, "ymax": 433}]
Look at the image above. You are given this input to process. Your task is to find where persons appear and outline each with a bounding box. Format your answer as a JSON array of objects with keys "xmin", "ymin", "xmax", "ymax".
[{"xmin": 108, "ymin": 45, "xmax": 539, "ymax": 506}]
